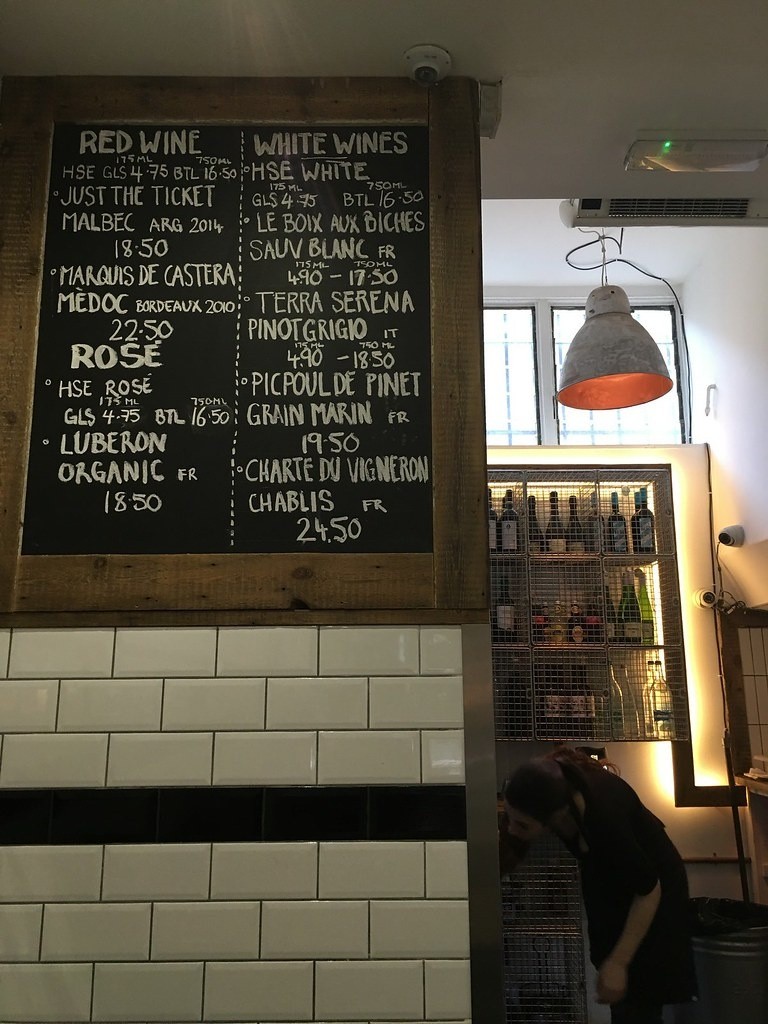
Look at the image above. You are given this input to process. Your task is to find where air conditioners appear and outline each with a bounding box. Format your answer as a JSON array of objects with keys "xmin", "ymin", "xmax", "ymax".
[{"xmin": 558, "ymin": 197, "xmax": 768, "ymax": 229}]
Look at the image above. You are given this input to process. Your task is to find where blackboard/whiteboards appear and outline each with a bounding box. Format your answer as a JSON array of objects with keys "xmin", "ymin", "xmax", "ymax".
[{"xmin": 0, "ymin": 73, "xmax": 487, "ymax": 630}]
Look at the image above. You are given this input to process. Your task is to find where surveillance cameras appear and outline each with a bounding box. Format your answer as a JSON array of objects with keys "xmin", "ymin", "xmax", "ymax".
[
  {"xmin": 692, "ymin": 589, "xmax": 718, "ymax": 610},
  {"xmin": 400, "ymin": 44, "xmax": 451, "ymax": 88},
  {"xmin": 718, "ymin": 524, "xmax": 744, "ymax": 548}
]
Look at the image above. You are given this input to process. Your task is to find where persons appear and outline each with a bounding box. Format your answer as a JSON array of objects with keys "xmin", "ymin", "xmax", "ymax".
[{"xmin": 500, "ymin": 744, "xmax": 701, "ymax": 1024}]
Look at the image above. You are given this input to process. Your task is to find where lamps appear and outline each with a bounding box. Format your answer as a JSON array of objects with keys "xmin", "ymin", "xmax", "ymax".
[{"xmin": 554, "ymin": 225, "xmax": 674, "ymax": 410}]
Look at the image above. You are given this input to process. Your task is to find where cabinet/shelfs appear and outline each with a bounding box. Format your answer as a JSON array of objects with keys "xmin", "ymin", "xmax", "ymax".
[{"xmin": 486, "ymin": 464, "xmax": 690, "ymax": 747}]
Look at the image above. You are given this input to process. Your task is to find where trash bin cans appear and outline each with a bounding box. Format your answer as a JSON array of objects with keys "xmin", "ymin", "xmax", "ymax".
[{"xmin": 663, "ymin": 896, "xmax": 768, "ymax": 1024}]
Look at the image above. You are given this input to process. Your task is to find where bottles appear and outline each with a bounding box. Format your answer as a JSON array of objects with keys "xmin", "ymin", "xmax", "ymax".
[
  {"xmin": 641, "ymin": 660, "xmax": 677, "ymax": 739},
  {"xmin": 543, "ymin": 664, "xmax": 566, "ymax": 735},
  {"xmin": 487, "ymin": 488, "xmax": 499, "ymax": 553},
  {"xmin": 587, "ymin": 597, "xmax": 601, "ymax": 643},
  {"xmin": 528, "ymin": 495, "xmax": 545, "ymax": 552},
  {"xmin": 608, "ymin": 660, "xmax": 641, "ymax": 739},
  {"xmin": 501, "ymin": 665, "xmax": 531, "ymax": 737},
  {"xmin": 565, "ymin": 496, "xmax": 585, "ymax": 551},
  {"xmin": 631, "ymin": 488, "xmax": 656, "ymax": 555},
  {"xmin": 532, "ymin": 600, "xmax": 586, "ymax": 643},
  {"xmin": 494, "ymin": 576, "xmax": 517, "ymax": 642},
  {"xmin": 545, "ymin": 491, "xmax": 566, "ymax": 551},
  {"xmin": 638, "ymin": 574, "xmax": 654, "ymax": 644},
  {"xmin": 569, "ymin": 664, "xmax": 594, "ymax": 736},
  {"xmin": 607, "ymin": 492, "xmax": 628, "ymax": 553},
  {"xmin": 586, "ymin": 492, "xmax": 606, "ymax": 553},
  {"xmin": 606, "ymin": 585, "xmax": 616, "ymax": 643},
  {"xmin": 617, "ymin": 571, "xmax": 642, "ymax": 642},
  {"xmin": 499, "ymin": 489, "xmax": 520, "ymax": 553}
]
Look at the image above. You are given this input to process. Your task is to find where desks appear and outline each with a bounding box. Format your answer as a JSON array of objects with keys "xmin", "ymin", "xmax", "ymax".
[{"xmin": 734, "ymin": 774, "xmax": 768, "ymax": 905}]
